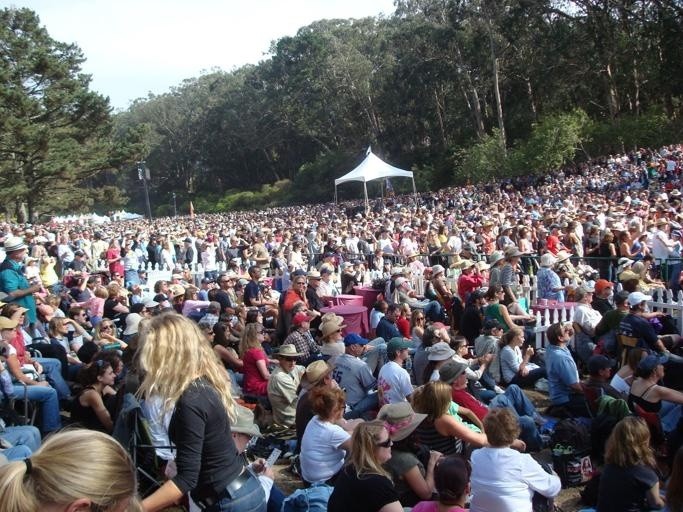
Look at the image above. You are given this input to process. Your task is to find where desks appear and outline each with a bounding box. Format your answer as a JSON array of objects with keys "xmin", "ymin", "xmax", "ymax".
[
  {"xmin": 319, "ymin": 294, "xmax": 364, "ymax": 308},
  {"xmin": 319, "ymin": 303, "xmax": 369, "ymax": 336},
  {"xmin": 351, "ymin": 285, "xmax": 385, "ymax": 324},
  {"xmin": 528, "ymin": 300, "xmax": 576, "ymax": 341}
]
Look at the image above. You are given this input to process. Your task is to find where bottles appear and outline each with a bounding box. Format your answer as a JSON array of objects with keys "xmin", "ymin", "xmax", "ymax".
[{"xmin": 101, "ymin": 343, "xmax": 120, "ymax": 351}]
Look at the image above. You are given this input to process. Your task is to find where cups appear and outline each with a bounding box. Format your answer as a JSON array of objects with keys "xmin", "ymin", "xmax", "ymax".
[
  {"xmin": 537, "ymin": 299, "xmax": 547, "ymax": 305},
  {"xmin": 358, "ymin": 283, "xmax": 361, "ymax": 289},
  {"xmin": 328, "ymin": 301, "xmax": 333, "ymax": 308}
]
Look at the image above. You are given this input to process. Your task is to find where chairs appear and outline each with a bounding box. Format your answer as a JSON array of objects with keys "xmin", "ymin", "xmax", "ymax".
[
  {"xmin": 580, "ymin": 383, "xmax": 606, "ymax": 420},
  {"xmin": 126, "ymin": 409, "xmax": 179, "ymax": 507},
  {"xmin": 1, "ymin": 346, "xmax": 42, "ymax": 426}
]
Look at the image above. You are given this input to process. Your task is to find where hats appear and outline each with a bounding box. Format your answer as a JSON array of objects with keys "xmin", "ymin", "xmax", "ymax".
[{"xmin": 0, "ymin": 183, "xmax": 680, "ymax": 442}]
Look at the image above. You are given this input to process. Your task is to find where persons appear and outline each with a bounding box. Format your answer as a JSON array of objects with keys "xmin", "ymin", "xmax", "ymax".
[{"xmin": 0, "ymin": 143, "xmax": 682, "ymax": 512}]
